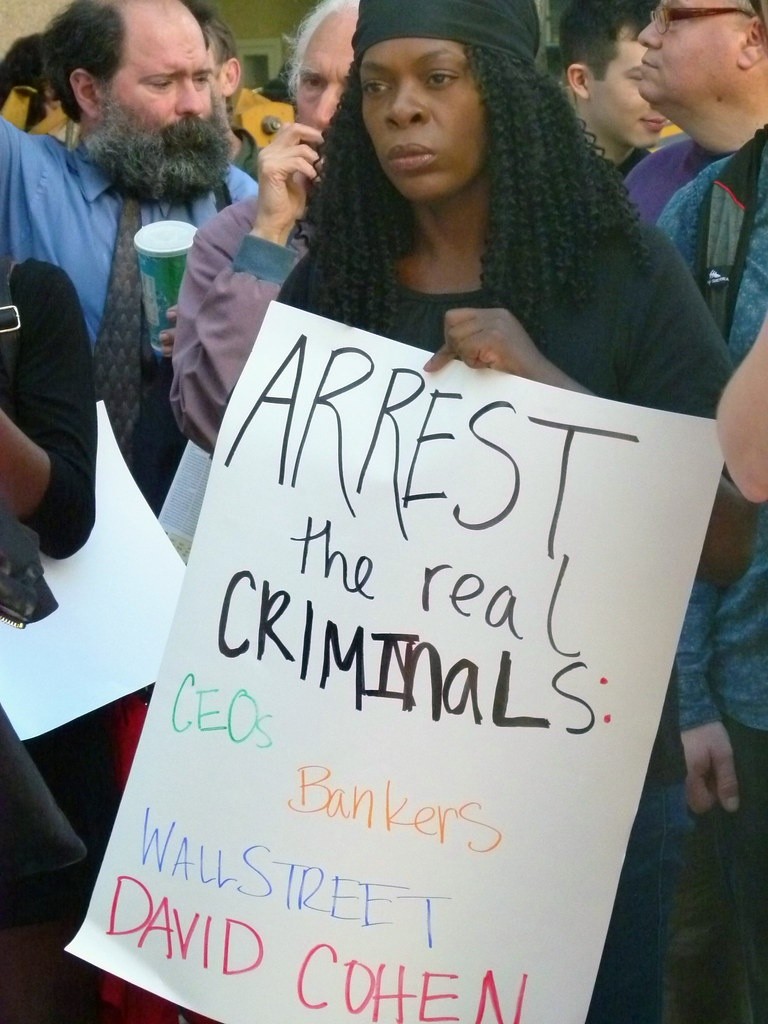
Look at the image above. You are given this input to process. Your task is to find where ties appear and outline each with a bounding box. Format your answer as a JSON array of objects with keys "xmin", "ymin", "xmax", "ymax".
[{"xmin": 93, "ymin": 185, "xmax": 144, "ymax": 474}]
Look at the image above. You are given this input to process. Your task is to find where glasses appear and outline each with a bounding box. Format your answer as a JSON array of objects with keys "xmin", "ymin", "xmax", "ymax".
[{"xmin": 648, "ymin": 6, "xmax": 760, "ymax": 36}]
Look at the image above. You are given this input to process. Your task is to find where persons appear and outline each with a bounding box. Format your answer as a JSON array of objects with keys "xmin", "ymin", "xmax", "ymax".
[
  {"xmin": 560, "ymin": 0, "xmax": 768, "ymax": 1024},
  {"xmin": 275, "ymin": 0, "xmax": 732, "ymax": 1024},
  {"xmin": 0, "ymin": 0, "xmax": 359, "ymax": 1024}
]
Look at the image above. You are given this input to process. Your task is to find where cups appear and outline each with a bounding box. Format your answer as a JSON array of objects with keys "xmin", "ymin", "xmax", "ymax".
[{"xmin": 133, "ymin": 220, "xmax": 198, "ymax": 352}]
